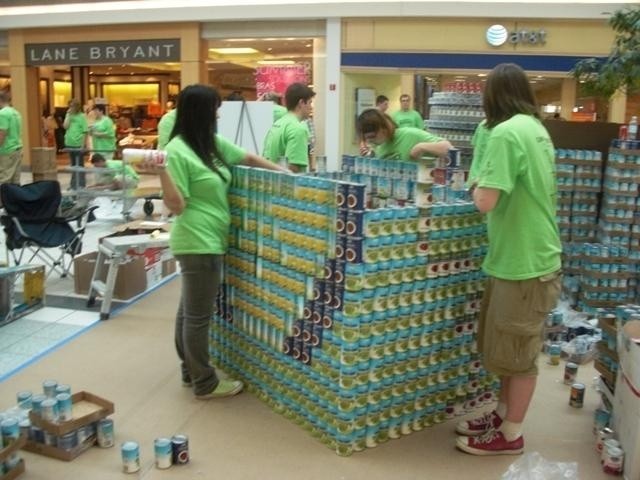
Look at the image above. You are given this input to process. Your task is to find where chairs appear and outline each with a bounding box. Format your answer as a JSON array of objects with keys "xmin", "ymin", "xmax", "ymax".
[{"xmin": 0, "ymin": 179, "xmax": 96, "ymax": 280}]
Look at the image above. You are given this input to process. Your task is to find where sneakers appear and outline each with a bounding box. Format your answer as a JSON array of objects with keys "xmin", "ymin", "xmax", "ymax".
[
  {"xmin": 455, "ymin": 409, "xmax": 505, "ymax": 435},
  {"xmin": 182, "ymin": 378, "xmax": 244, "ymax": 400},
  {"xmin": 456, "ymin": 430, "xmax": 525, "ymax": 455}
]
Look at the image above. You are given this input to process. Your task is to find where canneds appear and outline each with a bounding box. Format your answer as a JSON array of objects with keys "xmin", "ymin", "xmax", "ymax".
[
  {"xmin": 445, "ymin": 81, "xmax": 482, "ymax": 91},
  {"xmin": 209, "ymin": 149, "xmax": 500, "ymax": 456},
  {"xmin": 596, "ymin": 427, "xmax": 615, "ymax": 453},
  {"xmin": 598, "ymin": 304, "xmax": 639, "ymax": 391},
  {"xmin": 121, "ymin": 442, "xmax": 140, "ymax": 473},
  {"xmin": 121, "ymin": 147, "xmax": 165, "ymax": 165},
  {"xmin": 549, "ymin": 345, "xmax": 561, "ymax": 365},
  {"xmin": 601, "ymin": 439, "xmax": 620, "ymax": 465},
  {"xmin": 619, "ymin": 125, "xmax": 629, "ymax": 139},
  {"xmin": 1, "ymin": 379, "xmax": 115, "ymax": 478},
  {"xmin": 173, "ymin": 436, "xmax": 188, "ymax": 464},
  {"xmin": 569, "ymin": 383, "xmax": 585, "ymax": 408},
  {"xmin": 564, "ymin": 363, "xmax": 578, "ymax": 384},
  {"xmin": 603, "ymin": 448, "xmax": 625, "ymax": 474},
  {"xmin": 555, "ymin": 141, "xmax": 638, "ymax": 315},
  {"xmin": 593, "ymin": 409, "xmax": 611, "ymax": 435},
  {"xmin": 154, "ymin": 438, "xmax": 172, "ymax": 469},
  {"xmin": 545, "ymin": 309, "xmax": 562, "ymax": 328}
]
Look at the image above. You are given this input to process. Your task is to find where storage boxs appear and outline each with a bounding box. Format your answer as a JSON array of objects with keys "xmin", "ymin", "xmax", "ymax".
[
  {"xmin": 72, "ymin": 220, "xmax": 175, "ymax": 299},
  {"xmin": 594, "ymin": 315, "xmax": 640, "ymax": 480}
]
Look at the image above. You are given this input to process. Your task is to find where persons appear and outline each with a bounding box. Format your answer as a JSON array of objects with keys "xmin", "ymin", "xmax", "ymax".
[
  {"xmin": 133, "ymin": 83, "xmax": 296, "ymax": 400},
  {"xmin": 157, "ymin": 95, "xmax": 178, "ymax": 151},
  {"xmin": 356, "ymin": 108, "xmax": 453, "ymax": 162},
  {"xmin": 41, "ymin": 98, "xmax": 139, "ymax": 200},
  {"xmin": 226, "ymin": 91, "xmax": 246, "ymax": 101},
  {"xmin": 1, "ymin": 92, "xmax": 24, "ymax": 185},
  {"xmin": 390, "ymin": 94, "xmax": 427, "ymax": 129},
  {"xmin": 467, "ymin": 118, "xmax": 494, "ymax": 189},
  {"xmin": 374, "ymin": 94, "xmax": 390, "ymax": 112},
  {"xmin": 453, "ymin": 60, "xmax": 566, "ymax": 457}
]
